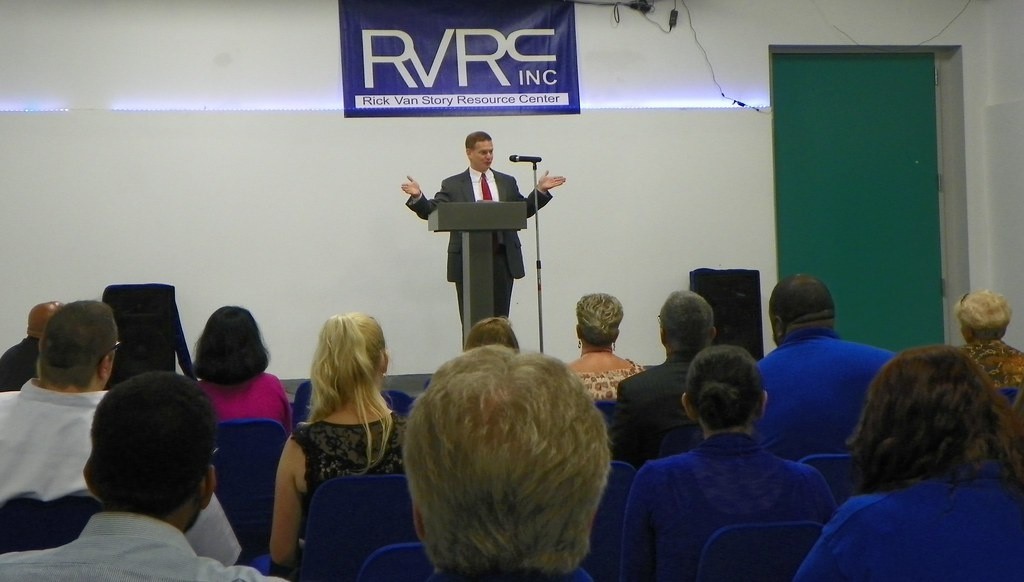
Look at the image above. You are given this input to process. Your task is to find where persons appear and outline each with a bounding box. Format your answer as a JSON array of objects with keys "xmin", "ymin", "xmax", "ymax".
[
  {"xmin": 0, "ymin": 275, "xmax": 1024, "ymax": 582},
  {"xmin": 401, "ymin": 131, "xmax": 566, "ymax": 353}
]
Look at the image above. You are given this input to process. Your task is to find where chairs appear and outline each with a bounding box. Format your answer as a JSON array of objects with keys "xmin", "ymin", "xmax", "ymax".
[{"xmin": 0, "ymin": 381, "xmax": 854, "ymax": 582}]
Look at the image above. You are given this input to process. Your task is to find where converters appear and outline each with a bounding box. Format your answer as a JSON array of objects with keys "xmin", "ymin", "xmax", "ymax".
[{"xmin": 669, "ymin": 10, "xmax": 678, "ymax": 26}]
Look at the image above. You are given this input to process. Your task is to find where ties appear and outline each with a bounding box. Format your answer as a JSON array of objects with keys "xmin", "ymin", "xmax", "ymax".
[{"xmin": 481, "ymin": 173, "xmax": 493, "ymax": 200}]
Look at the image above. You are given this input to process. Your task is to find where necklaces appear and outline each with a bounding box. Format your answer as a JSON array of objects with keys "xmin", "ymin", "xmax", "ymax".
[{"xmin": 580, "ymin": 350, "xmax": 610, "ymax": 357}]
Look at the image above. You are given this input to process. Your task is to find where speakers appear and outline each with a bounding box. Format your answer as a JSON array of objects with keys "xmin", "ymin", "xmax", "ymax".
[
  {"xmin": 102, "ymin": 284, "xmax": 176, "ymax": 387},
  {"xmin": 689, "ymin": 268, "xmax": 764, "ymax": 361}
]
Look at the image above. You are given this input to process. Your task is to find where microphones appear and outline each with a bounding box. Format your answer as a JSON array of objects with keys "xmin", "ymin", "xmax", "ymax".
[{"xmin": 509, "ymin": 155, "xmax": 541, "ymax": 162}]
